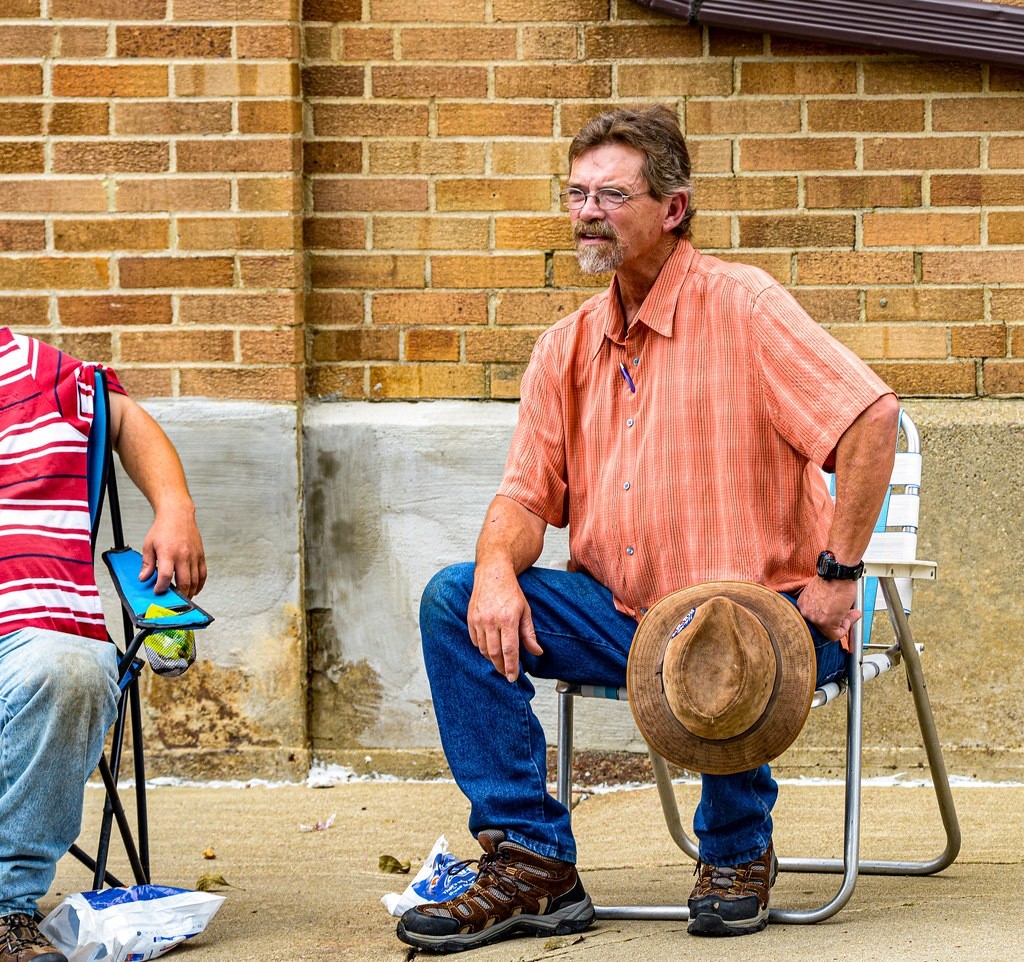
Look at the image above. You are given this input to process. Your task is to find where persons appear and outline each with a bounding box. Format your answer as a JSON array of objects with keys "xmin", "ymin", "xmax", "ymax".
[
  {"xmin": 393, "ymin": 105, "xmax": 901, "ymax": 955},
  {"xmin": 0, "ymin": 322, "xmax": 208, "ymax": 962}
]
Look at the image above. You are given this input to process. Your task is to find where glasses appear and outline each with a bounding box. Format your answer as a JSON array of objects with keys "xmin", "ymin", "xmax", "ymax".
[{"xmin": 557, "ymin": 186, "xmax": 652, "ymax": 211}]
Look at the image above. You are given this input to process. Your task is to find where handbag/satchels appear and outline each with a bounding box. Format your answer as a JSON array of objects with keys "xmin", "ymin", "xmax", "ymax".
[{"xmin": 36, "ymin": 883, "xmax": 228, "ymax": 962}]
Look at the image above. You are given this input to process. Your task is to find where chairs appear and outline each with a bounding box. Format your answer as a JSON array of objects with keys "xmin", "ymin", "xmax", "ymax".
[
  {"xmin": 555, "ymin": 410, "xmax": 961, "ymax": 923},
  {"xmin": 51, "ymin": 364, "xmax": 214, "ymax": 896}
]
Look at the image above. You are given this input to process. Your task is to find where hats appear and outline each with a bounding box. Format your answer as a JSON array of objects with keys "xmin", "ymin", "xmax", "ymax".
[{"xmin": 625, "ymin": 581, "xmax": 817, "ymax": 777}]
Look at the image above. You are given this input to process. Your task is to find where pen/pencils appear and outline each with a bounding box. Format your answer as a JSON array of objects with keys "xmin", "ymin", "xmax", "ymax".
[{"xmin": 619, "ymin": 363, "xmax": 635, "ymax": 393}]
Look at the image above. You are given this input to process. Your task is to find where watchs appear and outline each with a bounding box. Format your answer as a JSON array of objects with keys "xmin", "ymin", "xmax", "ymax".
[{"xmin": 816, "ymin": 551, "xmax": 865, "ymax": 581}]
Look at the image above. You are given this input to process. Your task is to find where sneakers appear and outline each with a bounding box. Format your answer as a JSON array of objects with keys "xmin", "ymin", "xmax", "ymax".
[
  {"xmin": 396, "ymin": 830, "xmax": 597, "ymax": 952},
  {"xmin": 687, "ymin": 836, "xmax": 779, "ymax": 933},
  {"xmin": 0, "ymin": 913, "xmax": 69, "ymax": 962}
]
